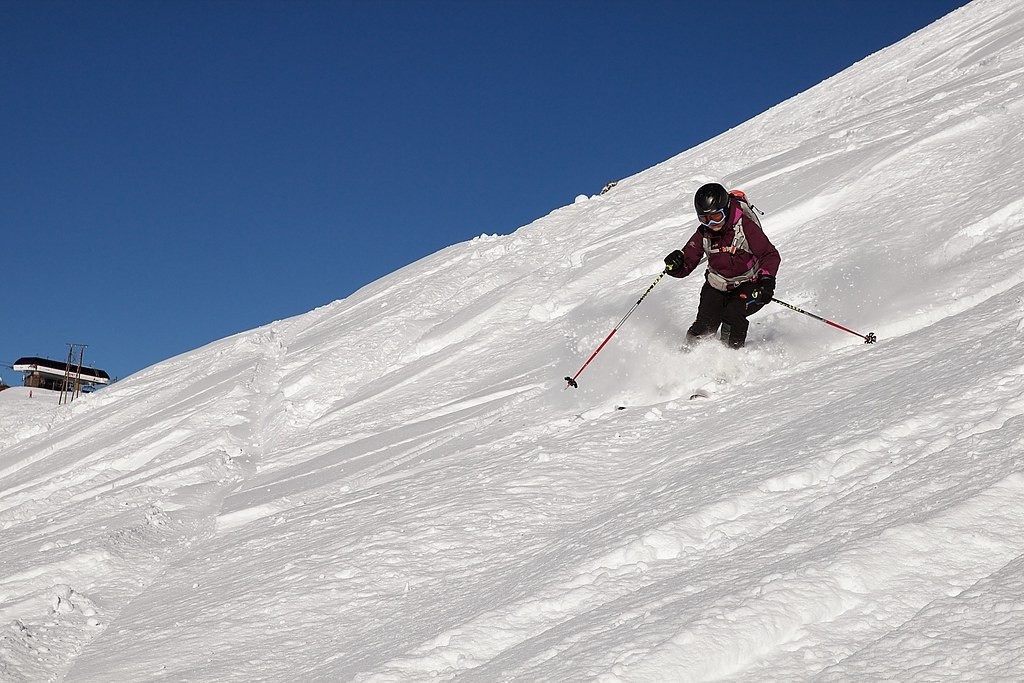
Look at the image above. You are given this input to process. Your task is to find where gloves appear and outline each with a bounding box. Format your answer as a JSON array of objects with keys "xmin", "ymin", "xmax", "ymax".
[
  {"xmin": 664, "ymin": 250, "xmax": 685, "ymax": 275},
  {"xmin": 750, "ymin": 278, "xmax": 774, "ymax": 304}
]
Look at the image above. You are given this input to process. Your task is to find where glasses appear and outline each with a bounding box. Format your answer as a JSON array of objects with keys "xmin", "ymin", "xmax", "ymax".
[{"xmin": 698, "ymin": 209, "xmax": 725, "ymax": 227}]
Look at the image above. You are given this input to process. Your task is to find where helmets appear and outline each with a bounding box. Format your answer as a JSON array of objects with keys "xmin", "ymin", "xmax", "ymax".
[{"xmin": 695, "ymin": 182, "xmax": 729, "ymax": 216}]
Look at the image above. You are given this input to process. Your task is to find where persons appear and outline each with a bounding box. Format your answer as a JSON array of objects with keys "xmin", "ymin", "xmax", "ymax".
[
  {"xmin": 664, "ymin": 182, "xmax": 782, "ymax": 354},
  {"xmin": 29, "ymin": 390, "xmax": 33, "ymax": 398}
]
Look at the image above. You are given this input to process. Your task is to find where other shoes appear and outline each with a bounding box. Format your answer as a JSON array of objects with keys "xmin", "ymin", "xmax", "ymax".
[
  {"xmin": 727, "ymin": 341, "xmax": 745, "ymax": 352},
  {"xmin": 681, "ymin": 331, "xmax": 705, "ymax": 352}
]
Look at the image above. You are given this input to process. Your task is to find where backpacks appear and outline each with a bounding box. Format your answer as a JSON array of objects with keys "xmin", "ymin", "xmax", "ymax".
[{"xmin": 703, "ymin": 190, "xmax": 765, "ymax": 259}]
